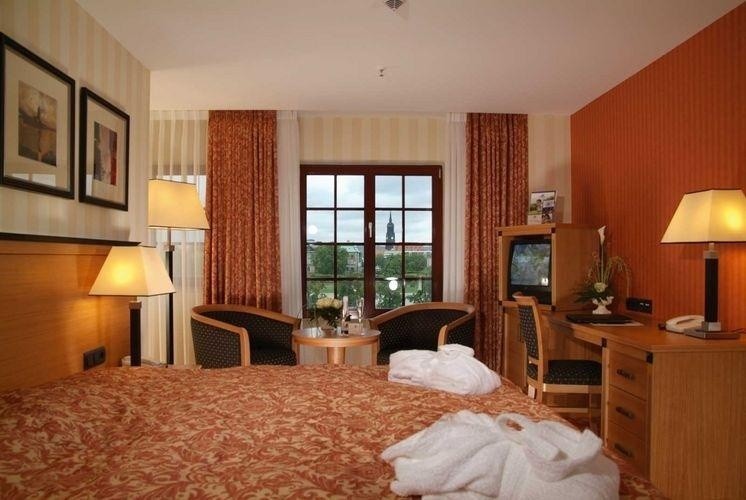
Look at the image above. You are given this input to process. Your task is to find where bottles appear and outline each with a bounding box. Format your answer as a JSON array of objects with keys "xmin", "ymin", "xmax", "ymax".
[{"xmin": 343, "ymin": 315, "xmax": 351, "ymax": 336}]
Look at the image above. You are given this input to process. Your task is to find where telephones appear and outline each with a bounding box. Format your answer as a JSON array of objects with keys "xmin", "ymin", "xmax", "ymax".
[{"xmin": 665, "ymin": 315, "xmax": 705, "ymax": 334}]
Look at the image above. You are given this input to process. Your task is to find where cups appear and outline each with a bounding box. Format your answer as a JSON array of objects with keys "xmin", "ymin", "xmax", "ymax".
[
  {"xmin": 121, "ymin": 356, "xmax": 130, "ymax": 367},
  {"xmin": 360, "ymin": 319, "xmax": 368, "ymax": 335}
]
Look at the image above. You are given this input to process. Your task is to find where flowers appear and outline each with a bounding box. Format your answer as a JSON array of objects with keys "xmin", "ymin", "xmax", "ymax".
[
  {"xmin": 306, "ymin": 295, "xmax": 346, "ymax": 328},
  {"xmin": 566, "ymin": 220, "xmax": 634, "ymax": 306}
]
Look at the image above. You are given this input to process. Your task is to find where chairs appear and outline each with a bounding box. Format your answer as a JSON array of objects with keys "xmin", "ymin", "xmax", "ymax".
[
  {"xmin": 507, "ymin": 288, "xmax": 604, "ymax": 433},
  {"xmin": 363, "ymin": 301, "xmax": 484, "ymax": 370},
  {"xmin": 186, "ymin": 301, "xmax": 303, "ymax": 374}
]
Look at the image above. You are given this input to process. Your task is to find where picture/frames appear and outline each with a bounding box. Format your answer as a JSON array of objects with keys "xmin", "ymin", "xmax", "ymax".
[
  {"xmin": 74, "ymin": 81, "xmax": 131, "ymax": 213},
  {"xmin": 0, "ymin": 26, "xmax": 78, "ymax": 204}
]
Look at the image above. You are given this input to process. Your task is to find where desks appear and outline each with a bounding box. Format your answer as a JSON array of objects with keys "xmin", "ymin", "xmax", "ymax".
[
  {"xmin": 499, "ymin": 297, "xmax": 745, "ymax": 500},
  {"xmin": 285, "ymin": 320, "xmax": 385, "ymax": 368}
]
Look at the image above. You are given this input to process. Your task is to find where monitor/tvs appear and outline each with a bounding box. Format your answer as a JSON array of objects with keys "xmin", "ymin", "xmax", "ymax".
[{"xmin": 507, "ymin": 239, "xmax": 552, "ymax": 306}]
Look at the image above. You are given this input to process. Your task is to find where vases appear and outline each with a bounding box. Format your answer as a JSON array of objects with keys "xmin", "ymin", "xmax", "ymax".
[
  {"xmin": 589, "ymin": 292, "xmax": 616, "ymax": 316},
  {"xmin": 318, "ymin": 315, "xmax": 338, "ymax": 337}
]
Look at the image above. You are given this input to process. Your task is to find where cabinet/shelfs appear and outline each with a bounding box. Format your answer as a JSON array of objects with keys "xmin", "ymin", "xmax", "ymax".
[{"xmin": 493, "ymin": 215, "xmax": 600, "ymax": 316}]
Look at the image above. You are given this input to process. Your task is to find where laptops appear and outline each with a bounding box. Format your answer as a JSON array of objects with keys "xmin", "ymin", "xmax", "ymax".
[{"xmin": 566, "ymin": 313, "xmax": 632, "ymax": 323}]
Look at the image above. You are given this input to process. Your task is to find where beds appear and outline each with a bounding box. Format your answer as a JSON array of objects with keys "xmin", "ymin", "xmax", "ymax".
[{"xmin": 0, "ymin": 351, "xmax": 671, "ymax": 500}]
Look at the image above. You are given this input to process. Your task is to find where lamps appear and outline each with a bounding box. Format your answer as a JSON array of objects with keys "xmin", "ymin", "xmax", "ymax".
[
  {"xmin": 87, "ymin": 243, "xmax": 178, "ymax": 369},
  {"xmin": 653, "ymin": 186, "xmax": 745, "ymax": 343},
  {"xmin": 143, "ymin": 177, "xmax": 210, "ymax": 366}
]
